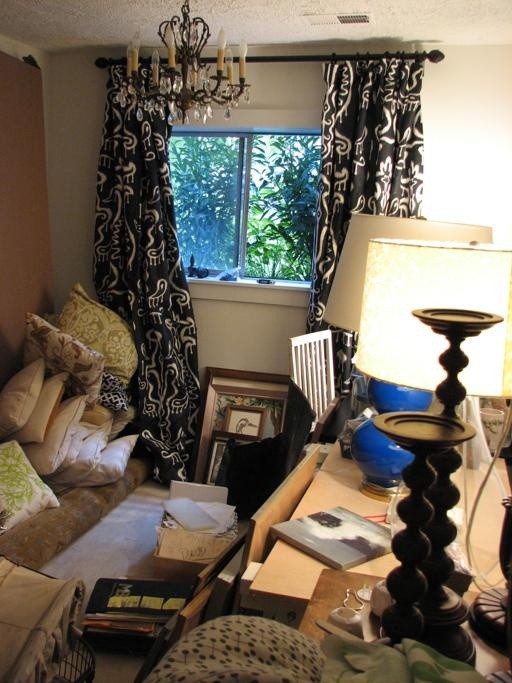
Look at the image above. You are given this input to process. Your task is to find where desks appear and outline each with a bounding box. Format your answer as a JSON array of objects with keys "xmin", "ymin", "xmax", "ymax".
[
  {"xmin": 298, "ymin": 569, "xmax": 511, "ymax": 678},
  {"xmin": 249, "ymin": 439, "xmax": 512, "ymax": 627}
]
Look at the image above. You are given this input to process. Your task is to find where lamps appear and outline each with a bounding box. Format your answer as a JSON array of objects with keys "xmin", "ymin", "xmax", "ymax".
[
  {"xmin": 322, "ymin": 215, "xmax": 493, "ymax": 503},
  {"xmin": 355, "ymin": 238, "xmax": 512, "ymax": 659},
  {"xmin": 126, "ymin": 0, "xmax": 252, "ymax": 127}
]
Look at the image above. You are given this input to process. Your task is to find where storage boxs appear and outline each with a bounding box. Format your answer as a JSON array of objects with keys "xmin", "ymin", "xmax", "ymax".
[{"xmin": 152, "ymin": 480, "xmax": 238, "ymax": 578}]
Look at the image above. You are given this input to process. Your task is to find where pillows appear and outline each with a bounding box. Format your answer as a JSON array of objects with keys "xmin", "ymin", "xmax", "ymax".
[{"xmin": 0, "ymin": 281, "xmax": 139, "ymax": 536}]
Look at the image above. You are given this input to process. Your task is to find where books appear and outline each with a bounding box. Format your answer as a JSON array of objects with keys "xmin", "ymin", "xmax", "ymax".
[{"xmin": 80, "ymin": 497, "xmax": 241, "ymax": 656}]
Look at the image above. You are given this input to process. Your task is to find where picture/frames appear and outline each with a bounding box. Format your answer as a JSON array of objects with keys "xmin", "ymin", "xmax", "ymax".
[{"xmin": 188, "ymin": 367, "xmax": 298, "ymax": 485}]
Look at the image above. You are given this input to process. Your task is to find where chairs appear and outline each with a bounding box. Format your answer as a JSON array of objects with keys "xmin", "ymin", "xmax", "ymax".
[{"xmin": 288, "ymin": 329, "xmax": 335, "ymax": 464}]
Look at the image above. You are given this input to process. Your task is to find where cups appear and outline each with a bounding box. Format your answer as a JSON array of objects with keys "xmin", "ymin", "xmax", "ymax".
[{"xmin": 475, "ymin": 408, "xmax": 504, "ymax": 459}]
